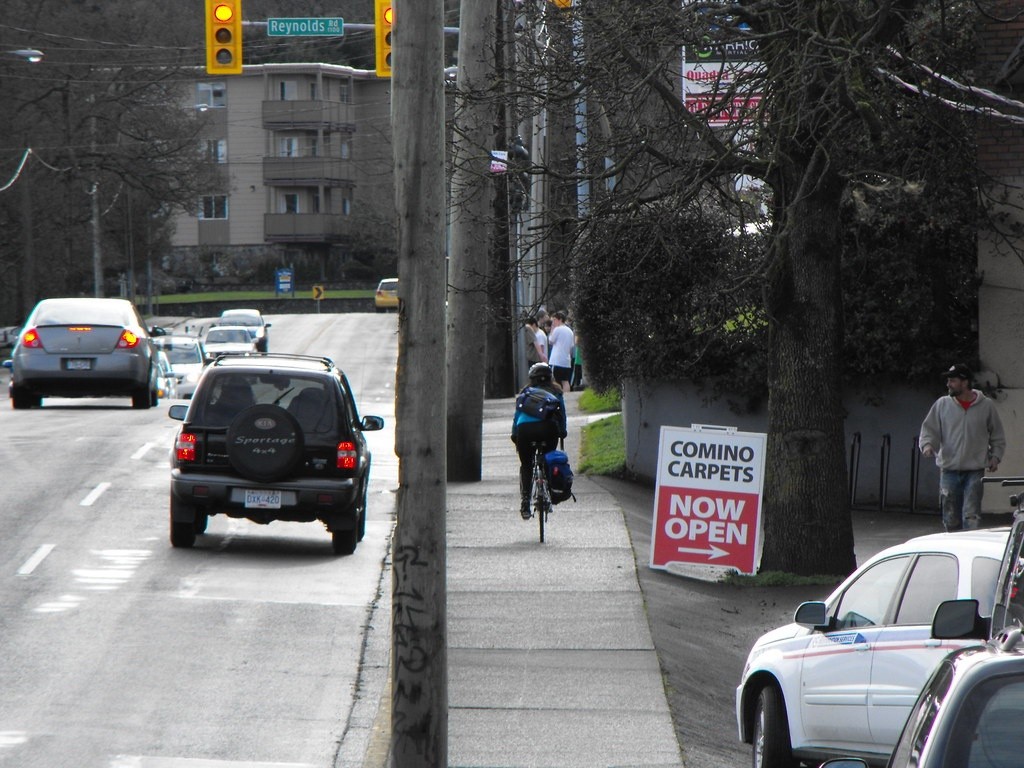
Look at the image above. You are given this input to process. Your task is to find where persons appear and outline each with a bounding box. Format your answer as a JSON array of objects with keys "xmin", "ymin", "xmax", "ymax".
[
  {"xmin": 548, "ymin": 313, "xmax": 574, "ymax": 393},
  {"xmin": 511, "ymin": 362, "xmax": 567, "ymax": 519},
  {"xmin": 536, "ymin": 310, "xmax": 549, "ymax": 333},
  {"xmin": 525, "ymin": 319, "xmax": 548, "ymax": 368},
  {"xmin": 560, "ymin": 309, "xmax": 582, "ymax": 386},
  {"xmin": 919, "ymin": 364, "xmax": 1005, "ymax": 533}
]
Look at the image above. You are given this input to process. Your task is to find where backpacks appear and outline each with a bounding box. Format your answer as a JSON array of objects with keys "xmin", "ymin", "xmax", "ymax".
[
  {"xmin": 545, "ymin": 449, "xmax": 574, "ymax": 505},
  {"xmin": 518, "ymin": 387, "xmax": 562, "ymax": 420}
]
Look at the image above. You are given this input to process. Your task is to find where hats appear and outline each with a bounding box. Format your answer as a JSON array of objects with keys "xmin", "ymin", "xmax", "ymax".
[
  {"xmin": 943, "ymin": 364, "xmax": 973, "ymax": 380},
  {"xmin": 537, "ymin": 310, "xmax": 549, "ymax": 320}
]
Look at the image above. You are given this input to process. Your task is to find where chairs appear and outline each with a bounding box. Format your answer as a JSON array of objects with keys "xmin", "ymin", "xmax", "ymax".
[
  {"xmin": 286, "ymin": 387, "xmax": 333, "ymax": 438},
  {"xmin": 207, "ymin": 378, "xmax": 254, "ymax": 427}
]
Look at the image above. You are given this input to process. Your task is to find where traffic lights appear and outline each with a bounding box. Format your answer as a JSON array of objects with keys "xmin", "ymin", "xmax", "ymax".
[
  {"xmin": 374, "ymin": 2, "xmax": 392, "ymax": 80},
  {"xmin": 206, "ymin": 0, "xmax": 243, "ymax": 75}
]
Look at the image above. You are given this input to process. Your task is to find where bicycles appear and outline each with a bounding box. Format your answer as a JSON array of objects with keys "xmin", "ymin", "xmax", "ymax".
[{"xmin": 510, "ymin": 433, "xmax": 564, "ymax": 543}]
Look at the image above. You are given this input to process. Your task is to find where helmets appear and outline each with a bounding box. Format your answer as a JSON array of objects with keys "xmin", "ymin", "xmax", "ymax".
[{"xmin": 528, "ymin": 362, "xmax": 552, "ymax": 378}]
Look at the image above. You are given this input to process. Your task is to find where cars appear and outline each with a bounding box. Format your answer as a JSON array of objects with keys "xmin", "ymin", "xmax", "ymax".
[
  {"xmin": 2, "ymin": 296, "xmax": 166, "ymax": 410},
  {"xmin": 732, "ymin": 472, "xmax": 1024, "ymax": 767},
  {"xmin": 0, "ymin": 325, "xmax": 22, "ymax": 348},
  {"xmin": 147, "ymin": 308, "xmax": 272, "ymax": 398},
  {"xmin": 373, "ymin": 277, "xmax": 400, "ymax": 313}
]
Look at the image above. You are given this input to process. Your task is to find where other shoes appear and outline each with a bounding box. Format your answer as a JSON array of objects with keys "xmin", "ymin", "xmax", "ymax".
[{"xmin": 521, "ymin": 500, "xmax": 531, "ymax": 519}]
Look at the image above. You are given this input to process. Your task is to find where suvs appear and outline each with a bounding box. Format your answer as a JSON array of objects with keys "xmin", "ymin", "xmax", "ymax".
[{"xmin": 167, "ymin": 350, "xmax": 386, "ymax": 555}]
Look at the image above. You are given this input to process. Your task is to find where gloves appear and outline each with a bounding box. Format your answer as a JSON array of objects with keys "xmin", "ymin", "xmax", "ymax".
[
  {"xmin": 559, "ymin": 431, "xmax": 567, "ymax": 438},
  {"xmin": 511, "ymin": 434, "xmax": 516, "ymax": 444}
]
